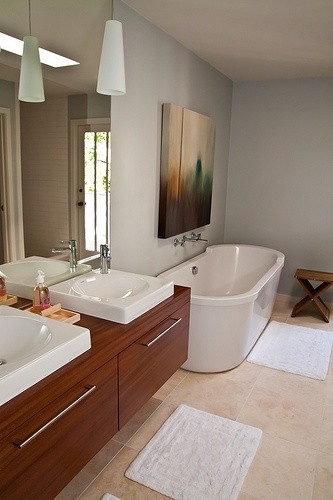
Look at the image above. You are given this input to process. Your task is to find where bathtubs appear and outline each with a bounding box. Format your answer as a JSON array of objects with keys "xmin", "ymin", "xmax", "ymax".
[{"xmin": 157, "ymin": 243, "xmax": 285, "ymax": 374}]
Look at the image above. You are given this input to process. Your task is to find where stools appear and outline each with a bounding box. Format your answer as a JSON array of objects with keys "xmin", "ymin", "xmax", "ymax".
[{"xmin": 291, "ymin": 269, "xmax": 333, "ymax": 323}]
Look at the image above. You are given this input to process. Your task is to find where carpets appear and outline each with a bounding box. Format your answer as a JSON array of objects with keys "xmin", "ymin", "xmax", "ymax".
[
  {"xmin": 124, "ymin": 404, "xmax": 264, "ymax": 500},
  {"xmin": 246, "ymin": 321, "xmax": 333, "ymax": 381}
]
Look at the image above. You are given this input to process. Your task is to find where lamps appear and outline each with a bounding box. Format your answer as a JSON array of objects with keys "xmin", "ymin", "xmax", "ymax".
[
  {"xmin": 97, "ymin": 0, "xmax": 126, "ymax": 96},
  {"xmin": 18, "ymin": 0, "xmax": 46, "ymax": 102}
]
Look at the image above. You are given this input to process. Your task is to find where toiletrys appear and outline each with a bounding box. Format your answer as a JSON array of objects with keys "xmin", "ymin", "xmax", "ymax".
[
  {"xmin": 0, "ymin": 271, "xmax": 7, "ymax": 301},
  {"xmin": 31, "ymin": 270, "xmax": 51, "ymax": 315}
]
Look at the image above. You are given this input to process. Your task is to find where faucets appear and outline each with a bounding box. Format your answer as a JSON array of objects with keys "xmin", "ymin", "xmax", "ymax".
[
  {"xmin": 99, "ymin": 244, "xmax": 112, "ymax": 275},
  {"xmin": 51, "ymin": 240, "xmax": 78, "ymax": 268},
  {"xmin": 181, "ymin": 233, "xmax": 209, "ymax": 245}
]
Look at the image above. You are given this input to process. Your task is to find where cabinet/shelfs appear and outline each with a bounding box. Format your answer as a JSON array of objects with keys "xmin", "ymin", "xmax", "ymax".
[{"xmin": 0, "ymin": 285, "xmax": 191, "ymax": 500}]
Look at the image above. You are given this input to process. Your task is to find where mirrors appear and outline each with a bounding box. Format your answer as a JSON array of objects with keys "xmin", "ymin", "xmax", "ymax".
[{"xmin": 0, "ymin": 0, "xmax": 112, "ymax": 271}]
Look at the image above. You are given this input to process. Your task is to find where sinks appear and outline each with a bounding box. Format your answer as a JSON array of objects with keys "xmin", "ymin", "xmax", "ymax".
[
  {"xmin": 0, "ymin": 305, "xmax": 94, "ymax": 405},
  {"xmin": 46, "ymin": 267, "xmax": 175, "ymax": 326},
  {"xmin": 1, "ymin": 255, "xmax": 92, "ymax": 300}
]
